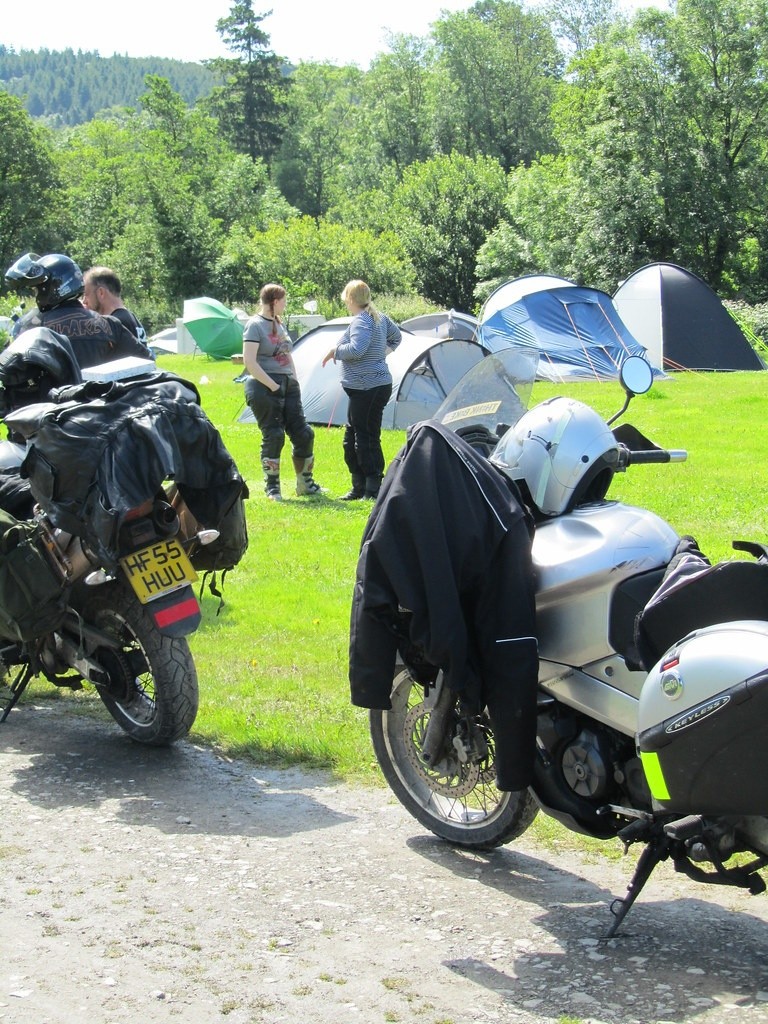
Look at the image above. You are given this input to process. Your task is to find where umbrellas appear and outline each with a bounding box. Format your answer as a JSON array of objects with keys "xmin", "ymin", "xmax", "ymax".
[{"xmin": 182, "ymin": 296, "xmax": 245, "ymax": 358}]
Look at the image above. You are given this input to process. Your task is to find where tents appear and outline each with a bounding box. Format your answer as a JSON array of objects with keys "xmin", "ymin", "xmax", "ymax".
[
  {"xmin": 234, "ymin": 316, "xmax": 529, "ymax": 430},
  {"xmin": 613, "ymin": 262, "xmax": 768, "ymax": 372},
  {"xmin": 399, "ymin": 307, "xmax": 553, "ymax": 382},
  {"xmin": 479, "ymin": 274, "xmax": 669, "ymax": 383}
]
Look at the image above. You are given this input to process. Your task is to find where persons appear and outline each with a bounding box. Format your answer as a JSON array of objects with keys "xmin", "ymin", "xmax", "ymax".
[
  {"xmin": 322, "ymin": 280, "xmax": 403, "ymax": 500},
  {"xmin": 81, "ymin": 267, "xmax": 146, "ymax": 347},
  {"xmin": 243, "ymin": 284, "xmax": 329, "ymax": 501},
  {"xmin": 4, "ymin": 253, "xmax": 155, "ymax": 370}
]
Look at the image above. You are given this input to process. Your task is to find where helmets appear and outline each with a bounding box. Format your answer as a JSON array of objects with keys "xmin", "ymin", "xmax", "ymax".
[
  {"xmin": 485, "ymin": 396, "xmax": 621, "ymax": 523},
  {"xmin": 30, "ymin": 255, "xmax": 87, "ymax": 313}
]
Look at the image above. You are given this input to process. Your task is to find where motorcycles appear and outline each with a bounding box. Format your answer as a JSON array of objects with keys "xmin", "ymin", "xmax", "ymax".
[
  {"xmin": 376, "ymin": 360, "xmax": 768, "ymax": 949},
  {"xmin": 0, "ymin": 328, "xmax": 217, "ymax": 746}
]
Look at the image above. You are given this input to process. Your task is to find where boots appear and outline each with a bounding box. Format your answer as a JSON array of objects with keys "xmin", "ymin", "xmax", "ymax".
[
  {"xmin": 338, "ymin": 472, "xmax": 366, "ymax": 500},
  {"xmin": 359, "ymin": 477, "xmax": 382, "ymax": 502},
  {"xmin": 259, "ymin": 456, "xmax": 282, "ymax": 503},
  {"xmin": 291, "ymin": 454, "xmax": 330, "ymax": 496}
]
